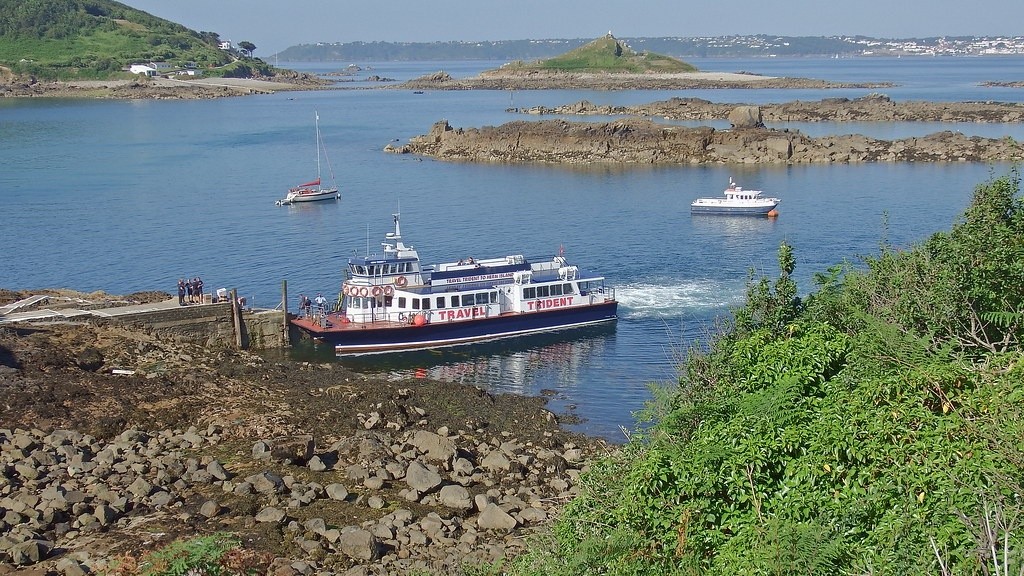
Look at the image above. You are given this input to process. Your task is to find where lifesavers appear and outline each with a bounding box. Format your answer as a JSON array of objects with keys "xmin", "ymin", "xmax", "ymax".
[
  {"xmin": 360, "ymin": 288, "xmax": 369, "ymax": 298},
  {"xmin": 372, "ymin": 287, "xmax": 383, "ymax": 296},
  {"xmin": 384, "ymin": 286, "xmax": 393, "ymax": 295},
  {"xmin": 398, "ymin": 276, "xmax": 407, "ymax": 286},
  {"xmin": 350, "ymin": 286, "xmax": 359, "ymax": 297}
]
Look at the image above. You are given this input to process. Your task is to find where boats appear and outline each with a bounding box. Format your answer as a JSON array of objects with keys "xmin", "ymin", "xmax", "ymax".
[
  {"xmin": 689, "ymin": 175, "xmax": 782, "ymax": 214},
  {"xmin": 287, "ymin": 196, "xmax": 621, "ymax": 356},
  {"xmin": 412, "ymin": 90, "xmax": 424, "ymax": 94}
]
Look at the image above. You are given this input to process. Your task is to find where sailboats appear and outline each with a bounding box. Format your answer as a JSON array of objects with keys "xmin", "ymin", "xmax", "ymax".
[{"xmin": 274, "ymin": 108, "xmax": 342, "ymax": 206}]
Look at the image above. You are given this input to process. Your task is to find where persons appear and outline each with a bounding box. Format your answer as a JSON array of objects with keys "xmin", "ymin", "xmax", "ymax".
[
  {"xmin": 315, "ymin": 294, "xmax": 328, "ymax": 312},
  {"xmin": 177, "ymin": 277, "xmax": 204, "ymax": 305},
  {"xmin": 305, "ymin": 297, "xmax": 312, "ymax": 316},
  {"xmin": 297, "ymin": 294, "xmax": 305, "ymax": 315}
]
